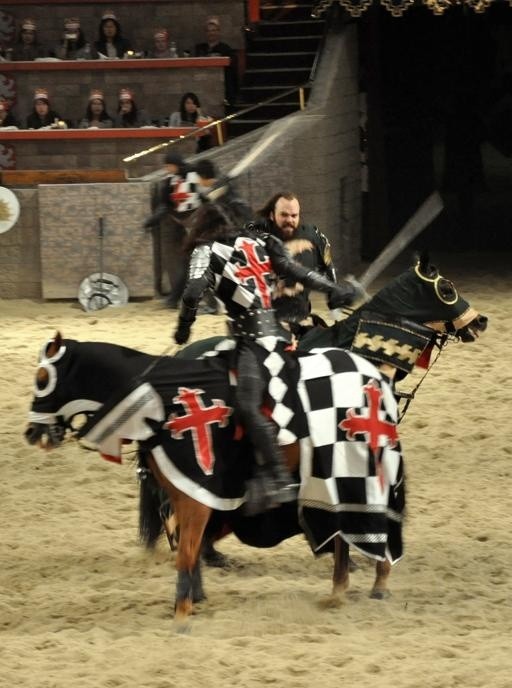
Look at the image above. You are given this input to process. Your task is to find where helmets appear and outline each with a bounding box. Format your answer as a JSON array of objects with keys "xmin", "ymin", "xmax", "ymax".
[{"xmin": 117, "ymin": 87, "xmax": 135, "ymax": 99}]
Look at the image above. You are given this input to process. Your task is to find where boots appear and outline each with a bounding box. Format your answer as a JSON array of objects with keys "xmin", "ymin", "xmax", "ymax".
[{"xmin": 246, "ymin": 423, "xmax": 303, "ymax": 496}]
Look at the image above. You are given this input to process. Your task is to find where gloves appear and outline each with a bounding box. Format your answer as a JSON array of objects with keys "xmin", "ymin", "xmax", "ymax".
[
  {"xmin": 173, "ymin": 318, "xmax": 192, "ymax": 346},
  {"xmin": 307, "ymin": 272, "xmax": 351, "ymax": 302}
]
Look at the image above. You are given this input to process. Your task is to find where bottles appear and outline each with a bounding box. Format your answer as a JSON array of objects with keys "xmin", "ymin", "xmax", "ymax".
[{"xmin": 170, "ymin": 40, "xmax": 178, "ymax": 58}]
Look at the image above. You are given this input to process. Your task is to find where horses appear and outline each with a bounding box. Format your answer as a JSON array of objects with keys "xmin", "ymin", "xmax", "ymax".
[
  {"xmin": 137, "ymin": 248, "xmax": 490, "ymax": 574},
  {"xmin": 24, "ymin": 330, "xmax": 408, "ymax": 620}
]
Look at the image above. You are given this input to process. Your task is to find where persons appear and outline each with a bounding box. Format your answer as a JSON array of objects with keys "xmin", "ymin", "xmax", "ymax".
[
  {"xmin": 194, "ymin": 14, "xmax": 239, "ymax": 108},
  {"xmin": 111, "ymin": 86, "xmax": 152, "ymax": 129},
  {"xmin": 167, "ymin": 91, "xmax": 215, "ymax": 131},
  {"xmin": 78, "ymin": 89, "xmax": 114, "ymax": 128},
  {"xmin": 54, "ymin": 16, "xmax": 93, "ymax": 61},
  {"xmin": 252, "ymin": 190, "xmax": 358, "ymax": 342},
  {"xmin": 91, "ymin": 8, "xmax": 132, "ymax": 60},
  {"xmin": 0, "ymin": 99, "xmax": 21, "ymax": 130},
  {"xmin": 144, "ymin": 26, "xmax": 179, "ymax": 59},
  {"xmin": 142, "ymin": 150, "xmax": 221, "ymax": 314},
  {"xmin": 190, "ymin": 158, "xmax": 258, "ymax": 225},
  {"xmin": 9, "ymin": 17, "xmax": 53, "ymax": 62},
  {"xmin": 173, "ymin": 203, "xmax": 294, "ymax": 515},
  {"xmin": 25, "ymin": 88, "xmax": 63, "ymax": 131}
]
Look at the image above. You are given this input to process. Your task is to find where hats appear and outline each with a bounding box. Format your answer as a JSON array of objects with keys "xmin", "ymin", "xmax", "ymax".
[
  {"xmin": 206, "ymin": 16, "xmax": 222, "ymax": 26},
  {"xmin": 33, "ymin": 87, "xmax": 51, "ymax": 99},
  {"xmin": 21, "ymin": 17, "xmax": 39, "ymax": 32},
  {"xmin": 88, "ymin": 88, "xmax": 104, "ymax": 101},
  {"xmin": 154, "ymin": 27, "xmax": 168, "ymax": 39},
  {"xmin": 63, "ymin": 18, "xmax": 79, "ymax": 28},
  {"xmin": 99, "ymin": 10, "xmax": 118, "ymax": 22}
]
